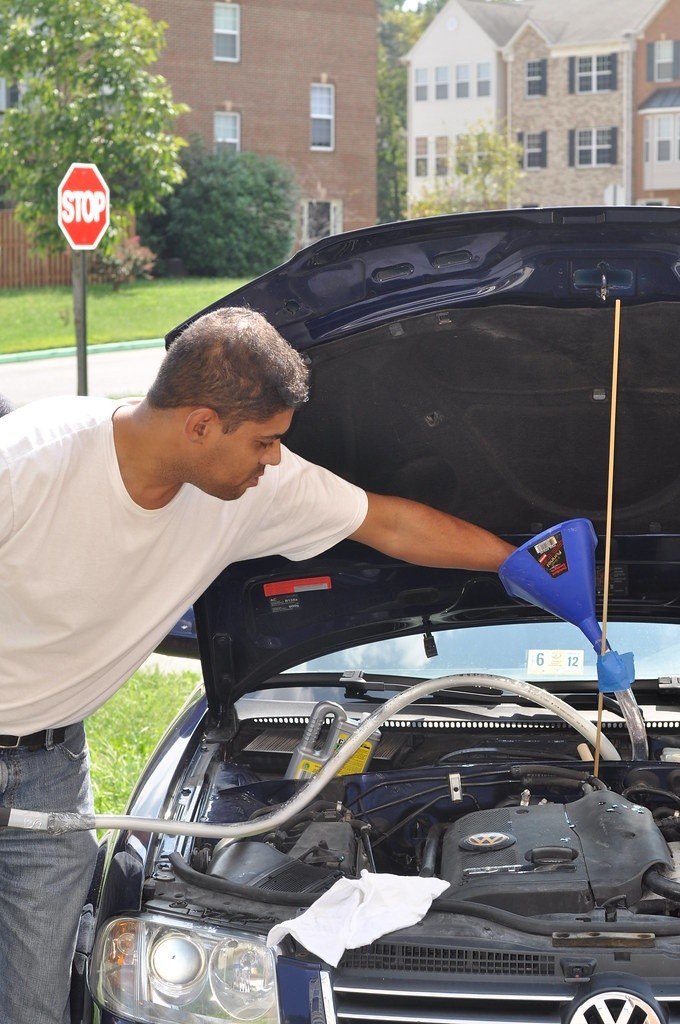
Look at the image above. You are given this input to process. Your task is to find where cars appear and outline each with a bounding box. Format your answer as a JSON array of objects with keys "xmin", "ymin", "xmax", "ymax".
[{"xmin": 69, "ymin": 205, "xmax": 679, "ymax": 1024}]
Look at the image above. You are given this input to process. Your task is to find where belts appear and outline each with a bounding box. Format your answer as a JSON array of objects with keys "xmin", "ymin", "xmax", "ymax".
[{"xmin": 0, "ymin": 728, "xmax": 65, "ymax": 749}]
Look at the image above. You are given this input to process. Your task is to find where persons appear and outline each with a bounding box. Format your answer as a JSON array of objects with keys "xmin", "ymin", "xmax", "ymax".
[{"xmin": 0, "ymin": 307, "xmax": 518, "ymax": 1024}]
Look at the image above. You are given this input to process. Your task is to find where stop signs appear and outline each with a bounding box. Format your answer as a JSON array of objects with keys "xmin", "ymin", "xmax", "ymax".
[{"xmin": 58, "ymin": 162, "xmax": 110, "ymax": 251}]
